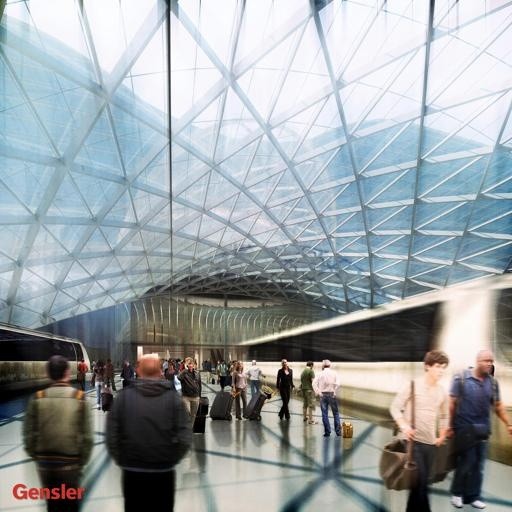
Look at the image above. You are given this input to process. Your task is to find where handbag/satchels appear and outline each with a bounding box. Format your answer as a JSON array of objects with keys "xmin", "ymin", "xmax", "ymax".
[
  {"xmin": 341, "ymin": 420, "xmax": 354, "ymax": 438},
  {"xmin": 426, "ymin": 424, "xmax": 491, "ymax": 485},
  {"xmin": 378, "ymin": 435, "xmax": 416, "ymax": 491}
]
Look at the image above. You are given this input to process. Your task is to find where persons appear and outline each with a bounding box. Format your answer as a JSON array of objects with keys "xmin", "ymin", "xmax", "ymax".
[
  {"xmin": 446, "ymin": 350, "xmax": 512, "ymax": 509},
  {"xmin": 389, "ymin": 350, "xmax": 451, "ymax": 512}
]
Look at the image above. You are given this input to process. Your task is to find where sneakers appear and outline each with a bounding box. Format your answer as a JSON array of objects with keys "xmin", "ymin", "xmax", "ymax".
[
  {"xmin": 336, "ymin": 431, "xmax": 342, "ymax": 436},
  {"xmin": 323, "ymin": 432, "xmax": 331, "ymax": 437},
  {"xmin": 470, "ymin": 499, "xmax": 485, "ymax": 509},
  {"xmin": 303, "ymin": 416, "xmax": 308, "ymax": 422},
  {"xmin": 449, "ymin": 494, "xmax": 464, "ymax": 509},
  {"xmin": 308, "ymin": 419, "xmax": 319, "ymax": 424},
  {"xmin": 277, "ymin": 412, "xmax": 292, "ymax": 420}
]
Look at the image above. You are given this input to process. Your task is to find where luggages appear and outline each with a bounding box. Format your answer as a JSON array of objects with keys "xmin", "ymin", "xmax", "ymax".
[
  {"xmin": 192, "ymin": 396, "xmax": 210, "ymax": 434},
  {"xmin": 101, "ymin": 391, "xmax": 115, "ymax": 412},
  {"xmin": 242, "ymin": 390, "xmax": 269, "ymax": 421},
  {"xmin": 209, "ymin": 390, "xmax": 234, "ymax": 422}
]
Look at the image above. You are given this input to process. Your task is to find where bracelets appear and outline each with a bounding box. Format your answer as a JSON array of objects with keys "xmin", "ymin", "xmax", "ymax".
[{"xmin": 505, "ymin": 425, "xmax": 511, "ymax": 426}]
